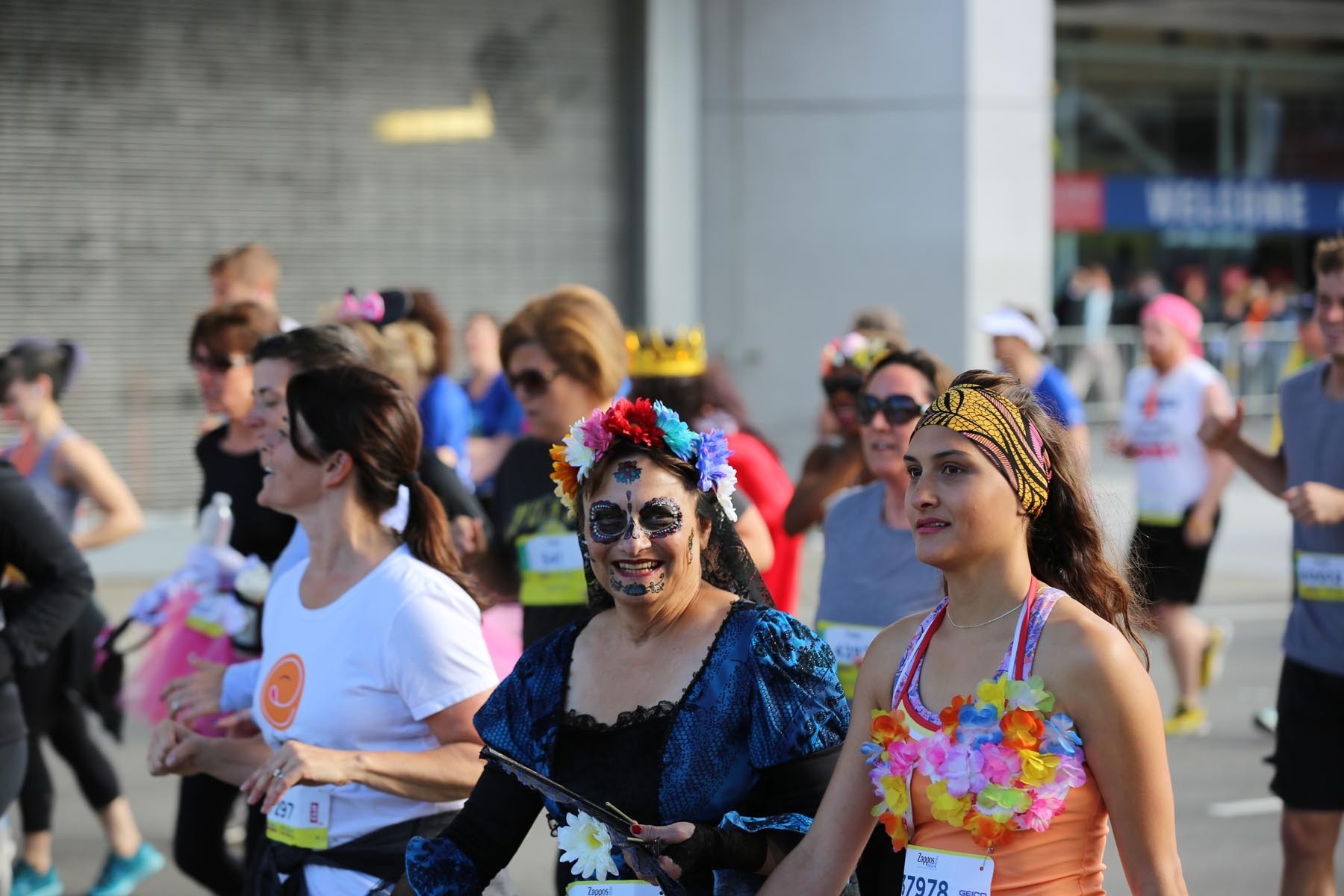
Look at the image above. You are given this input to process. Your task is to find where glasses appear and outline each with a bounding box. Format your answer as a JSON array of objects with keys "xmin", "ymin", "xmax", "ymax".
[
  {"xmin": 193, "ymin": 353, "xmax": 249, "ymax": 372},
  {"xmin": 853, "ymin": 394, "xmax": 930, "ymax": 424},
  {"xmin": 508, "ymin": 363, "xmax": 566, "ymax": 391},
  {"xmin": 823, "ymin": 376, "xmax": 861, "ymax": 395}
]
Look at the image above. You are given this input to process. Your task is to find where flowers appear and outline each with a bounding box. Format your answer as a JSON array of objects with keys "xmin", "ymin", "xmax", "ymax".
[
  {"xmin": 557, "ymin": 809, "xmax": 647, "ymax": 883},
  {"xmin": 550, "ymin": 395, "xmax": 738, "ymax": 524},
  {"xmin": 859, "ymin": 675, "xmax": 1087, "ymax": 853}
]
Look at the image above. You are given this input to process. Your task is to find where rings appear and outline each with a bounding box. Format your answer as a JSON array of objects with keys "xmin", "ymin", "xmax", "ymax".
[
  {"xmin": 273, "ymin": 768, "xmax": 285, "ymax": 780},
  {"xmin": 172, "ymin": 700, "xmax": 180, "ymax": 712}
]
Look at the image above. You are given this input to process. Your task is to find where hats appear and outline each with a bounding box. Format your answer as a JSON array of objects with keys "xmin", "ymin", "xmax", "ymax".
[{"xmin": 1139, "ymin": 292, "xmax": 1203, "ymax": 344}]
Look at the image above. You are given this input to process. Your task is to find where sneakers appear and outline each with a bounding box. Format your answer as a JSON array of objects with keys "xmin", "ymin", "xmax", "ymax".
[
  {"xmin": 88, "ymin": 840, "xmax": 167, "ymax": 896},
  {"xmin": 9, "ymin": 860, "xmax": 64, "ymax": 896}
]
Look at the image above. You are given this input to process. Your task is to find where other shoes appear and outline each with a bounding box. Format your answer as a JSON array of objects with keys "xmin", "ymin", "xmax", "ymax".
[
  {"xmin": 1200, "ymin": 620, "xmax": 1233, "ymax": 688},
  {"xmin": 1164, "ymin": 703, "xmax": 1206, "ymax": 737}
]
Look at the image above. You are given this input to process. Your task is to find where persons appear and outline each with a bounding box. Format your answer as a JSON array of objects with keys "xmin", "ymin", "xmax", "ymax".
[
  {"xmin": 452, "ymin": 281, "xmax": 1082, "ymax": 659},
  {"xmin": 754, "ymin": 372, "xmax": 1197, "ymax": 896},
  {"xmin": 369, "ymin": 402, "xmax": 903, "ymax": 896},
  {"xmin": 0, "ymin": 335, "xmax": 165, "ymax": 896},
  {"xmin": 144, "ymin": 243, "xmax": 500, "ymax": 896},
  {"xmin": 1200, "ymin": 234, "xmax": 1344, "ymax": 896},
  {"xmin": 1112, "ymin": 294, "xmax": 1241, "ymax": 740},
  {"xmin": 1052, "ymin": 267, "xmax": 1326, "ymax": 408}
]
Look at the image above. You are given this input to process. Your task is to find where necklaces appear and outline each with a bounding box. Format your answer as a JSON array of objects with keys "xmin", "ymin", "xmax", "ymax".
[{"xmin": 944, "ymin": 605, "xmax": 1025, "ymax": 629}]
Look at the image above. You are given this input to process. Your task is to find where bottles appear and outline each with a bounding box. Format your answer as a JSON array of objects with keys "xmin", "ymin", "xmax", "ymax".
[{"xmin": 197, "ymin": 491, "xmax": 234, "ymax": 545}]
